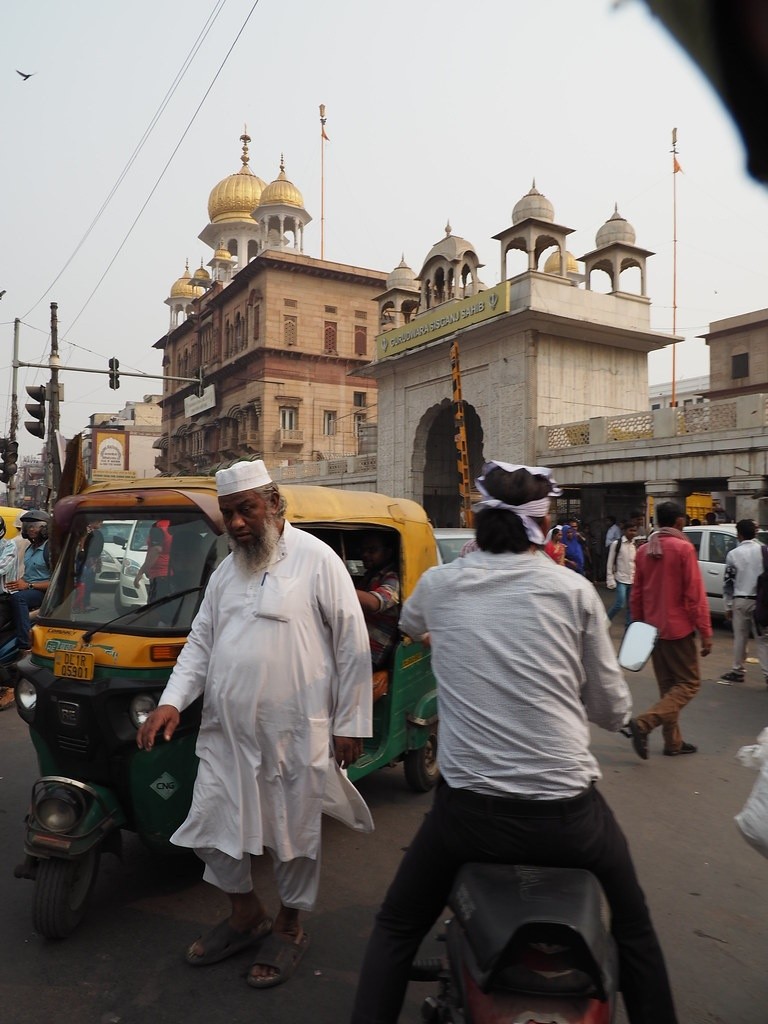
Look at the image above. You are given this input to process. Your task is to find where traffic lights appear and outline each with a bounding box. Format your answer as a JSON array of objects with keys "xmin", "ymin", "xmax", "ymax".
[
  {"xmin": 4, "ymin": 441, "xmax": 20, "ymax": 476},
  {"xmin": 24, "ymin": 384, "xmax": 47, "ymax": 439},
  {"xmin": 0, "ymin": 437, "xmax": 9, "ymax": 483}
]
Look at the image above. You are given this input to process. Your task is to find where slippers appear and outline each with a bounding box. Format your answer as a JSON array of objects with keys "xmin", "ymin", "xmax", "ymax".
[
  {"xmin": 630, "ymin": 718, "xmax": 648, "ymax": 760},
  {"xmin": 665, "ymin": 741, "xmax": 696, "ymax": 755},
  {"xmin": 186, "ymin": 917, "xmax": 273, "ymax": 965},
  {"xmin": 247, "ymin": 931, "xmax": 309, "ymax": 987}
]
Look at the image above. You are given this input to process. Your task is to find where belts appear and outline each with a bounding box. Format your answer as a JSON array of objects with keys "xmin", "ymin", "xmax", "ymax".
[
  {"xmin": 453, "ymin": 792, "xmax": 594, "ymax": 819},
  {"xmin": 737, "ymin": 596, "xmax": 757, "ymax": 599}
]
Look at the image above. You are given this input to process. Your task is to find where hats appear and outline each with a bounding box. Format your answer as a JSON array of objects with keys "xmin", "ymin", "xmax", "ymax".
[
  {"xmin": 214, "ymin": 460, "xmax": 272, "ymax": 496},
  {"xmin": 344, "ymin": 530, "xmax": 400, "ymax": 552}
]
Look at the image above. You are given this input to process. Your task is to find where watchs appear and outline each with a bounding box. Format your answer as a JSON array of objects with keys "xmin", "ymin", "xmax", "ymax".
[{"xmin": 29, "ymin": 582, "xmax": 34, "ymax": 590}]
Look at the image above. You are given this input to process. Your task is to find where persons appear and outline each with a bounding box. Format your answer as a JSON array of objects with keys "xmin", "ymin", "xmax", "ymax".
[
  {"xmin": 719, "ymin": 518, "xmax": 768, "ymax": 689},
  {"xmin": 137, "ymin": 460, "xmax": 373, "ymax": 989},
  {"xmin": 351, "ymin": 534, "xmax": 401, "ymax": 703},
  {"xmin": 543, "ymin": 512, "xmax": 646, "ymax": 591},
  {"xmin": 683, "ymin": 510, "xmax": 718, "ymax": 527},
  {"xmin": 349, "ymin": 460, "xmax": 678, "ymax": 1024},
  {"xmin": 605, "ymin": 521, "xmax": 636, "ymax": 627},
  {"xmin": 627, "ymin": 502, "xmax": 713, "ymax": 758},
  {"xmin": 0, "ymin": 509, "xmax": 104, "ymax": 712},
  {"xmin": 134, "ymin": 518, "xmax": 174, "ymax": 601}
]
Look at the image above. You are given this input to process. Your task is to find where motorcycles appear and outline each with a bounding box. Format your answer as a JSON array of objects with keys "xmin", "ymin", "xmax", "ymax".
[{"xmin": 8, "ymin": 475, "xmax": 438, "ymax": 945}]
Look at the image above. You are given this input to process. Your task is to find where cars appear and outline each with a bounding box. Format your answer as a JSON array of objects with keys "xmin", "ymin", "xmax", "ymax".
[
  {"xmin": 93, "ymin": 519, "xmax": 136, "ymax": 590},
  {"xmin": 113, "ymin": 519, "xmax": 157, "ymax": 617},
  {"xmin": 432, "ymin": 528, "xmax": 560, "ymax": 567}
]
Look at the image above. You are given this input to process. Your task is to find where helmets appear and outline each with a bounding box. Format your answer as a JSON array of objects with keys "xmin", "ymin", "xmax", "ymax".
[
  {"xmin": 0, "ymin": 516, "xmax": 6, "ymax": 540},
  {"xmin": 20, "ymin": 510, "xmax": 50, "ymax": 540}
]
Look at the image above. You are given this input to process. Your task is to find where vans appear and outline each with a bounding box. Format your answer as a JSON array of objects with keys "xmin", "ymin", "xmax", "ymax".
[{"xmin": 677, "ymin": 523, "xmax": 768, "ymax": 616}]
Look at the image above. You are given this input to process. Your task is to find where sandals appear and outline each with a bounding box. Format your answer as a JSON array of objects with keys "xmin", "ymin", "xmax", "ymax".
[{"xmin": 721, "ymin": 671, "xmax": 745, "ymax": 682}]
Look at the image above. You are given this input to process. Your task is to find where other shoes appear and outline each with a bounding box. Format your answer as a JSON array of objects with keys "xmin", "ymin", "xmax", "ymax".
[
  {"xmin": 0, "ymin": 689, "xmax": 15, "ymax": 710},
  {"xmin": 84, "ymin": 604, "xmax": 96, "ymax": 611},
  {"xmin": 11, "ymin": 648, "xmax": 32, "ymax": 669}
]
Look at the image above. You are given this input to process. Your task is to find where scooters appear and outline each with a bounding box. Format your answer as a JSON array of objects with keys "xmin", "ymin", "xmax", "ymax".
[
  {"xmin": 409, "ymin": 617, "xmax": 661, "ymax": 1024},
  {"xmin": 0, "ymin": 603, "xmax": 47, "ymax": 688}
]
